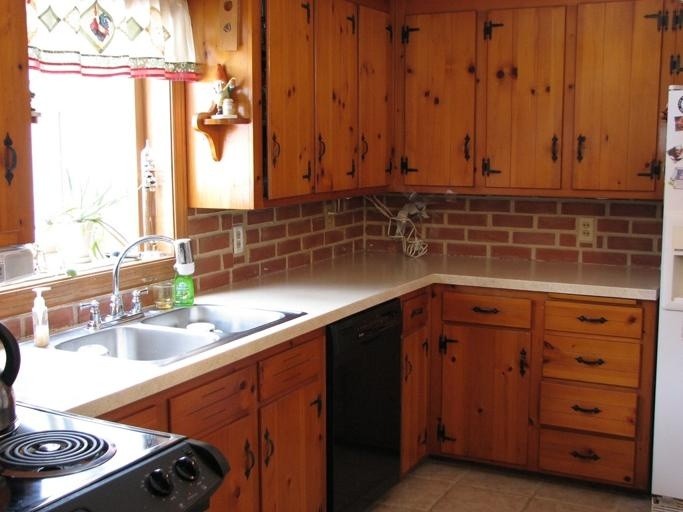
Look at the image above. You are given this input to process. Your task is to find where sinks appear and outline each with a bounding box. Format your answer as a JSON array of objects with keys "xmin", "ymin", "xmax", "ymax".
[
  {"xmin": 16, "ymin": 322, "xmax": 222, "ymax": 369},
  {"xmin": 124, "ymin": 299, "xmax": 309, "ymax": 338}
]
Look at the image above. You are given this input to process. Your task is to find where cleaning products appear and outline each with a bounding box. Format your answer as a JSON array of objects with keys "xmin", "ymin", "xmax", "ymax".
[
  {"xmin": 32, "ymin": 286, "xmax": 53, "ymax": 348},
  {"xmin": 168, "ymin": 271, "xmax": 195, "ymax": 307}
]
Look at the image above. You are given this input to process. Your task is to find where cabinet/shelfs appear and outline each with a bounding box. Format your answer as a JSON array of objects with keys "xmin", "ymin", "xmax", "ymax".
[
  {"xmin": 403, "ymin": 10, "xmax": 477, "ymax": 191},
  {"xmin": 440, "ymin": 290, "xmax": 534, "ymax": 468},
  {"xmin": 539, "ymin": 300, "xmax": 645, "ymax": 490},
  {"xmin": 402, "ymin": 294, "xmax": 433, "ymax": 472},
  {"xmin": 0, "ymin": 0, "xmax": 38, "ymax": 250},
  {"xmin": 254, "ymin": 0, "xmax": 393, "ymax": 201},
  {"xmin": 486, "ymin": 0, "xmax": 664, "ymax": 194}
]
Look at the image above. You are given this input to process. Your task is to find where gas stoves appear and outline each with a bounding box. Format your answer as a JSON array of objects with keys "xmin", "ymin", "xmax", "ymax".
[{"xmin": 1, "ymin": 398, "xmax": 230, "ymax": 509}]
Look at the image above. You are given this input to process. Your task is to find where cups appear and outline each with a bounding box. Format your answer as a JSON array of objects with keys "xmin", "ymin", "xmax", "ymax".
[
  {"xmin": 186, "ymin": 321, "xmax": 223, "ymax": 334},
  {"xmin": 152, "ymin": 283, "xmax": 174, "ymax": 308}
]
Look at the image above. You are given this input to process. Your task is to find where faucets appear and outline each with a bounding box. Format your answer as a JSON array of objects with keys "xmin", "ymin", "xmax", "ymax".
[{"xmin": 110, "ymin": 234, "xmax": 195, "ymax": 317}]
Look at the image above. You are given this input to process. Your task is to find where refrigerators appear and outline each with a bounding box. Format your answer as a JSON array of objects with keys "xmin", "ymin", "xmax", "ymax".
[{"xmin": 650, "ymin": 84, "xmax": 683, "ymax": 498}]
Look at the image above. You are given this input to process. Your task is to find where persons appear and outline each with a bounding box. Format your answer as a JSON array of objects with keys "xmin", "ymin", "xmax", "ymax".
[{"xmin": 668, "ymin": 145, "xmax": 683, "ymax": 162}]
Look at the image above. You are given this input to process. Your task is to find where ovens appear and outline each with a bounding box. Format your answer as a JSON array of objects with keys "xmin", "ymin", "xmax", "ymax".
[{"xmin": 326, "ymin": 296, "xmax": 402, "ymax": 512}]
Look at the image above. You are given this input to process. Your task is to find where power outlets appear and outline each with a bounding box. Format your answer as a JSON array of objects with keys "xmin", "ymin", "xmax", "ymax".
[{"xmin": 575, "ymin": 215, "xmax": 597, "ymax": 245}]
